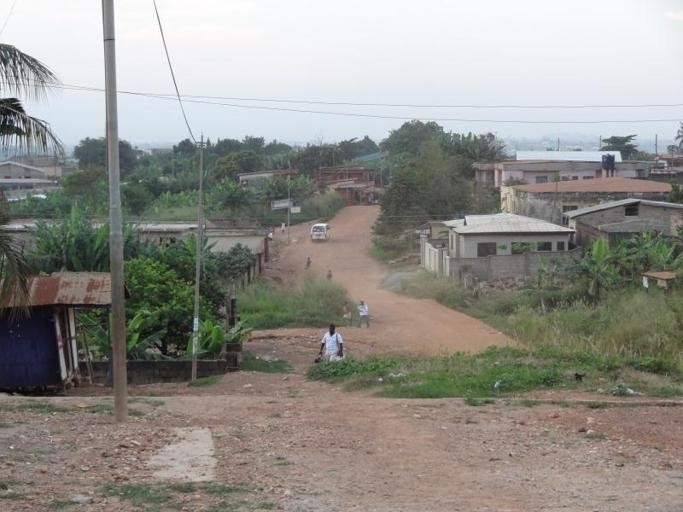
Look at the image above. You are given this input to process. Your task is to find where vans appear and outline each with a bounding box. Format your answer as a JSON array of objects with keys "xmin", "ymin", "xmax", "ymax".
[{"xmin": 308, "ymin": 223, "xmax": 333, "ymax": 241}]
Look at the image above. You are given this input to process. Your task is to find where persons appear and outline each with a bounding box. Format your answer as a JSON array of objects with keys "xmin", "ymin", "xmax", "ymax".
[
  {"xmin": 327, "ymin": 269, "xmax": 332, "ymax": 280},
  {"xmin": 306, "ymin": 256, "xmax": 311, "ymax": 271},
  {"xmin": 342, "ymin": 300, "xmax": 353, "ymax": 327},
  {"xmin": 319, "ymin": 322, "xmax": 344, "ymax": 361},
  {"xmin": 358, "ymin": 301, "xmax": 370, "ymax": 328}
]
